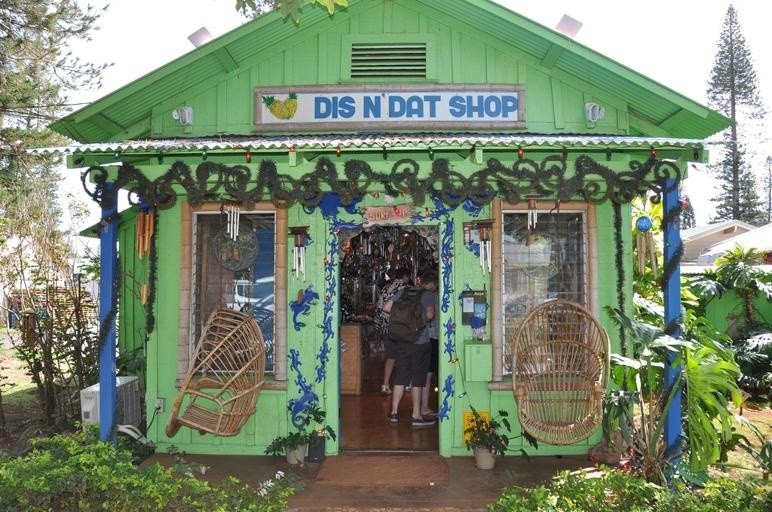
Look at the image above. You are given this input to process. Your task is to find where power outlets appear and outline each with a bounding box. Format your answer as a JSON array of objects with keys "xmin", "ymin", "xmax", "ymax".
[{"xmin": 156, "ymin": 397, "xmax": 164, "ymax": 413}]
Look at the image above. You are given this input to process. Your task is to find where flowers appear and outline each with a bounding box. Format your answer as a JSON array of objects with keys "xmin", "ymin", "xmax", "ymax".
[{"xmin": 464, "ymin": 405, "xmax": 538, "ymax": 462}]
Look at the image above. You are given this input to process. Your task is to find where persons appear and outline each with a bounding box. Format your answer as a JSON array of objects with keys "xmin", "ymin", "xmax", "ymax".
[{"xmin": 374, "ymin": 267, "xmax": 439, "ymax": 425}]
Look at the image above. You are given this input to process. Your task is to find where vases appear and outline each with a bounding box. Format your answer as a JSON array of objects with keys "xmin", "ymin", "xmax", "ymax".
[{"xmin": 474, "ymin": 445, "xmax": 497, "ymax": 470}]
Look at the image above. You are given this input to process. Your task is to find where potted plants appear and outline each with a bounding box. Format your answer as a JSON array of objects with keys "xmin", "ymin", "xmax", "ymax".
[
  {"xmin": 264, "ymin": 402, "xmax": 326, "ymax": 465},
  {"xmin": 306, "ymin": 425, "xmax": 336, "ymax": 462}
]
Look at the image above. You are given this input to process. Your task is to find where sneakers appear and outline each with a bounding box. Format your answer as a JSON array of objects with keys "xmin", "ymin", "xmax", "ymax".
[
  {"xmin": 410, "ymin": 412, "xmax": 439, "ymax": 426},
  {"xmin": 387, "ymin": 412, "xmax": 399, "ymax": 422},
  {"xmin": 405, "ymin": 384, "xmax": 411, "ymax": 392},
  {"xmin": 382, "ymin": 385, "xmax": 392, "ymax": 395}
]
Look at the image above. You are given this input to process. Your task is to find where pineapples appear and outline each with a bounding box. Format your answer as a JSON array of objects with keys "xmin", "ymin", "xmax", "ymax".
[
  {"xmin": 262, "ymin": 96, "xmax": 290, "ymax": 119},
  {"xmin": 284, "ymin": 93, "xmax": 298, "ymax": 118}
]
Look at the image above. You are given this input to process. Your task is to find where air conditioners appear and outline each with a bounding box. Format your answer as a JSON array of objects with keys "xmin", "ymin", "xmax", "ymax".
[{"xmin": 80, "ymin": 376, "xmax": 141, "ymax": 443}]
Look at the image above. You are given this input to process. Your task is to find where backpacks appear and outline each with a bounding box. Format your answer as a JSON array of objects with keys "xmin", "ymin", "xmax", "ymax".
[{"xmin": 389, "ymin": 286, "xmax": 427, "ymax": 344}]
